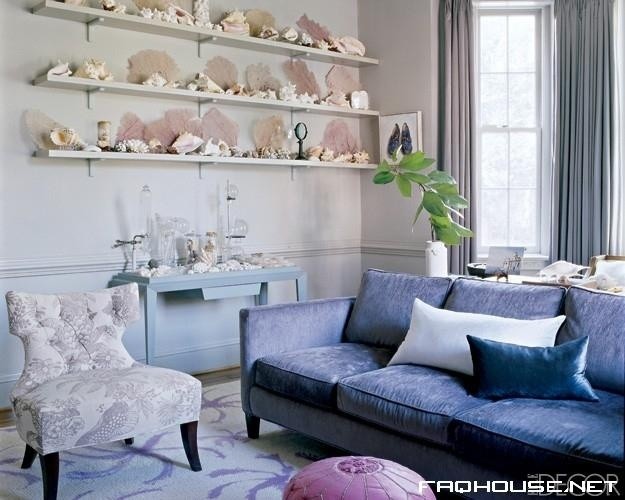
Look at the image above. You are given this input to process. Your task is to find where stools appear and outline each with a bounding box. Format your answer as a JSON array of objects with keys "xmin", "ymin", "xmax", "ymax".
[{"xmin": 283, "ymin": 453, "xmax": 437, "ymax": 499}]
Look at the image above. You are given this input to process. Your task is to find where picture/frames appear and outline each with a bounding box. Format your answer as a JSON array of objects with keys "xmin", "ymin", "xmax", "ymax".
[{"xmin": 378, "ymin": 111, "xmax": 424, "ymax": 167}]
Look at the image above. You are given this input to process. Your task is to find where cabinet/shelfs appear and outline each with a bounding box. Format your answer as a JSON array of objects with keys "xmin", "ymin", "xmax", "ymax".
[{"xmin": 27, "ymin": 2, "xmax": 378, "ymax": 180}]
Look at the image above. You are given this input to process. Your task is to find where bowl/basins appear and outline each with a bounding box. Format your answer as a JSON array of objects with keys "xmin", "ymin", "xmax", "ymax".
[{"xmin": 467, "ymin": 263, "xmax": 505, "ymax": 278}]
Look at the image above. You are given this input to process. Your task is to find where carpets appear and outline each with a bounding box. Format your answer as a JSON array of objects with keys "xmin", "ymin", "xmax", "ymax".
[{"xmin": 0, "ymin": 373, "xmax": 330, "ymax": 500}]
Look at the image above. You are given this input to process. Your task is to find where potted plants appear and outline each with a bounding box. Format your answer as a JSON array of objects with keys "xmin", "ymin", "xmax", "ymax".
[{"xmin": 368, "ymin": 143, "xmax": 474, "ymax": 278}]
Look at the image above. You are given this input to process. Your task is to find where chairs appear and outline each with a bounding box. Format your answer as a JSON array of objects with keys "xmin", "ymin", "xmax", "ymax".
[{"xmin": 5, "ymin": 282, "xmax": 205, "ymax": 499}]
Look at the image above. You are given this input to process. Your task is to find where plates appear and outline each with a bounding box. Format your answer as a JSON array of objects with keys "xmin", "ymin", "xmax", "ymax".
[{"xmin": 341, "ymin": 35, "xmax": 366, "ymax": 59}]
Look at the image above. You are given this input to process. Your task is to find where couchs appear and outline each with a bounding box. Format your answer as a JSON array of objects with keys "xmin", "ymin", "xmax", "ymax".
[{"xmin": 236, "ymin": 292, "xmax": 625, "ymax": 500}]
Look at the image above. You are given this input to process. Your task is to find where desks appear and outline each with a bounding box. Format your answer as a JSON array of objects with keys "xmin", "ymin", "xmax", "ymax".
[{"xmin": 108, "ymin": 263, "xmax": 311, "ymax": 375}]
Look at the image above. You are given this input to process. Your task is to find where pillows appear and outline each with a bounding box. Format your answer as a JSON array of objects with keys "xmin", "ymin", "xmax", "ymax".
[
  {"xmin": 554, "ymin": 285, "xmax": 625, "ymax": 396},
  {"xmin": 441, "ymin": 277, "xmax": 564, "ymax": 324},
  {"xmin": 382, "ymin": 299, "xmax": 567, "ymax": 378},
  {"xmin": 466, "ymin": 332, "xmax": 598, "ymax": 402},
  {"xmin": 345, "ymin": 267, "xmax": 453, "ymax": 355}
]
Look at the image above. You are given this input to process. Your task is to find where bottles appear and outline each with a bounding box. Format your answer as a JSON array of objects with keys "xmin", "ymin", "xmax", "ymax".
[
  {"xmin": 97, "ymin": 120, "xmax": 111, "ymax": 151},
  {"xmin": 204, "ymin": 231, "xmax": 218, "ymax": 267},
  {"xmin": 184, "ymin": 229, "xmax": 202, "ymax": 264},
  {"xmin": 161, "ymin": 216, "xmax": 179, "ymax": 266}
]
